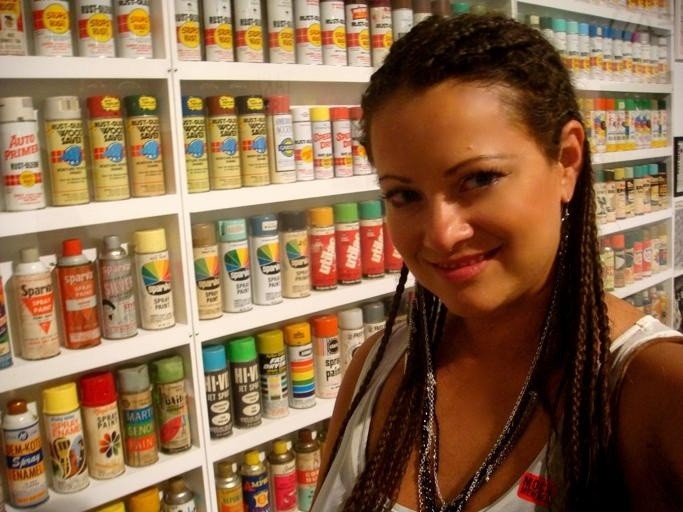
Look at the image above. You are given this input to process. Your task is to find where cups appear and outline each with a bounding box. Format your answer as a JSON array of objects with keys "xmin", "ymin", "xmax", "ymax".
[{"xmin": 0, "ymin": 1, "xmax": 153, "ymax": 60}]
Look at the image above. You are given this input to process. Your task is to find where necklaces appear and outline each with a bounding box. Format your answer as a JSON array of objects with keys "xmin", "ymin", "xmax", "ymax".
[{"xmin": 418, "ymin": 231, "xmax": 570, "ymax": 510}]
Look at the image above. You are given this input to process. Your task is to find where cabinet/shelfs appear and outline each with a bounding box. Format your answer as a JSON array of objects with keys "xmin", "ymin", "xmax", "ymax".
[
  {"xmin": 163, "ymin": 2, "xmax": 514, "ymax": 512},
  {"xmin": 0, "ymin": 1, "xmax": 210, "ymax": 511},
  {"xmin": 513, "ymin": 1, "xmax": 675, "ymax": 334}
]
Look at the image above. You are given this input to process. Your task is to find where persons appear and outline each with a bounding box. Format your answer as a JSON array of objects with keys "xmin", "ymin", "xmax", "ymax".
[{"xmin": 310, "ymin": 11, "xmax": 683, "ymax": 510}]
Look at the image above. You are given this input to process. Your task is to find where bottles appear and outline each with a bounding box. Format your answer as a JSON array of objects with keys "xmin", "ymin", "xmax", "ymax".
[
  {"xmin": 383, "ymin": 295, "xmax": 406, "ymax": 327},
  {"xmin": 624, "ymin": 287, "xmax": 671, "ymax": 325},
  {"xmin": 212, "ymin": 429, "xmax": 336, "ymax": 510},
  {"xmin": 40, "ymin": 380, "xmax": 90, "ymax": 495},
  {"xmin": 382, "ymin": 199, "xmax": 406, "ymax": 274},
  {"xmin": 348, "ymin": 107, "xmax": 372, "ymax": 176},
  {"xmin": 1, "ymin": 398, "xmax": 49, "ymax": 508},
  {"xmin": 334, "ymin": 307, "xmax": 364, "ymax": 375},
  {"xmin": 216, "ymin": 218, "xmax": 253, "ymax": 314},
  {"xmin": 329, "ymin": 107, "xmax": 353, "ymax": 177},
  {"xmin": 0, "ymin": 96, "xmax": 47, "ymax": 212},
  {"xmin": 333, "ymin": 203, "xmax": 363, "ymax": 286},
  {"xmin": 54, "ymin": 238, "xmax": 101, "ymax": 351},
  {"xmin": 84, "ymin": 94, "xmax": 130, "ymax": 202},
  {"xmin": 181, "ymin": 95, "xmax": 210, "ymax": 193},
  {"xmin": 517, "ymin": 12, "xmax": 669, "ymax": 86},
  {"xmin": 121, "ymin": 94, "xmax": 164, "ymax": 198},
  {"xmin": 11, "ymin": 246, "xmax": 61, "ymax": 361},
  {"xmin": 281, "ymin": 319, "xmax": 317, "ymax": 409},
  {"xmin": 41, "ymin": 95, "xmax": 89, "ymax": 206},
  {"xmin": 77, "ymin": 370, "xmax": 125, "ymax": 481},
  {"xmin": 131, "ymin": 228, "xmax": 175, "ymax": 330},
  {"xmin": 278, "ymin": 211, "xmax": 312, "ymax": 299},
  {"xmin": 254, "ymin": 330, "xmax": 289, "ymax": 419},
  {"xmin": 225, "ymin": 336, "xmax": 264, "ymax": 430},
  {"xmin": 150, "ymin": 355, "xmax": 192, "ymax": 454},
  {"xmin": 360, "ymin": 301, "xmax": 386, "ymax": 341},
  {"xmin": 160, "ymin": 476, "xmax": 196, "ymax": 511},
  {"xmin": 356, "ymin": 200, "xmax": 384, "ymax": 278},
  {"xmin": 176, "ymin": 0, "xmax": 503, "ymax": 67},
  {"xmin": 203, "ymin": 95, "xmax": 241, "ymax": 190},
  {"xmin": 289, "ymin": 106, "xmax": 315, "ymax": 181},
  {"xmin": 307, "ymin": 315, "xmax": 342, "ymax": 399},
  {"xmin": 191, "ymin": 222, "xmax": 223, "ymax": 320},
  {"xmin": 201, "ymin": 343, "xmax": 234, "ymax": 442},
  {"xmin": 306, "ymin": 206, "xmax": 338, "ymax": 292},
  {"xmin": 245, "ymin": 214, "xmax": 282, "ymax": 306},
  {"xmin": 595, "ymin": 225, "xmax": 672, "ymax": 291},
  {"xmin": 576, "ymin": 96, "xmax": 670, "ymax": 153},
  {"xmin": 95, "ymin": 234, "xmax": 138, "ymax": 340},
  {"xmin": 266, "ymin": 95, "xmax": 296, "ymax": 184},
  {"xmin": 309, "ymin": 106, "xmax": 334, "ymax": 179},
  {"xmin": 591, "ymin": 160, "xmax": 670, "ymax": 226},
  {"xmin": 234, "ymin": 95, "xmax": 270, "ymax": 187},
  {"xmin": 113, "ymin": 362, "xmax": 160, "ymax": 467}
]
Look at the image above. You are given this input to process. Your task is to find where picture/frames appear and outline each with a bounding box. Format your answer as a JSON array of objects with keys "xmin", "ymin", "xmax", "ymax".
[{"xmin": 673, "ymin": 136, "xmax": 683, "ymax": 198}]
